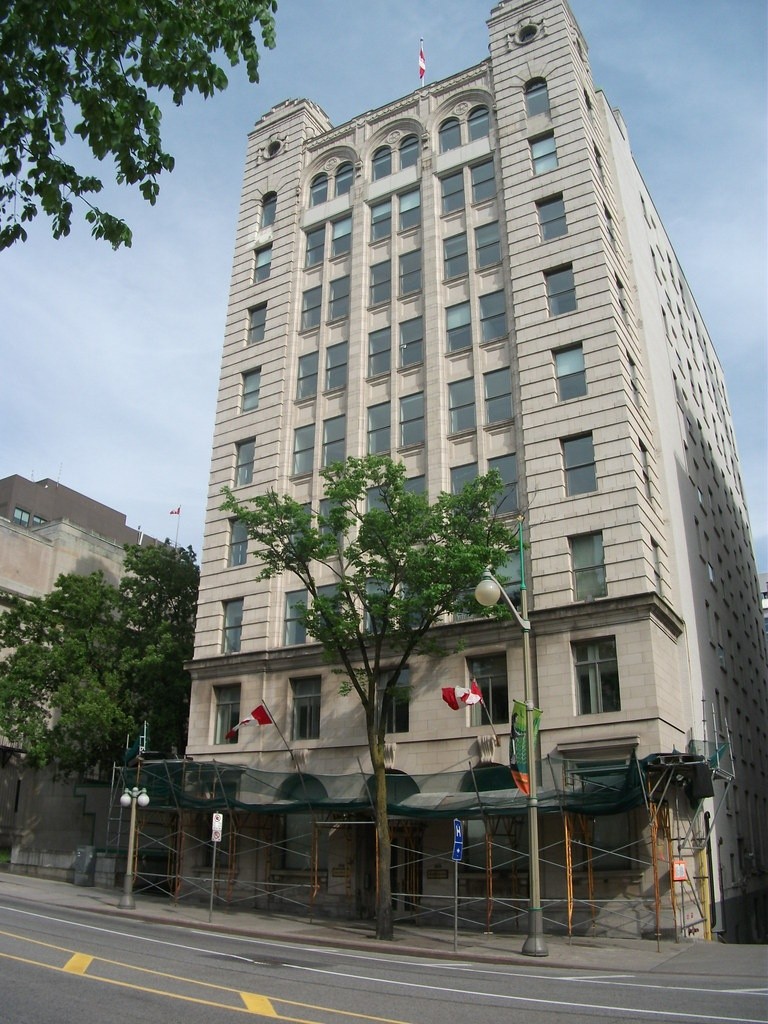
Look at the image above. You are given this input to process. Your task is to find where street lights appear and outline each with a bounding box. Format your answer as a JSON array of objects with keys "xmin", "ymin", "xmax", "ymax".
[
  {"xmin": 475, "ymin": 514, "xmax": 550, "ymax": 958},
  {"xmin": 116, "ymin": 786, "xmax": 151, "ymax": 910}
]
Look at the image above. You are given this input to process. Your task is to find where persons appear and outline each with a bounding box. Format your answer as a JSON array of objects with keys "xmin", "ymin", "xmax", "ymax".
[{"xmin": 510, "ymin": 710, "xmax": 526, "ymax": 770}]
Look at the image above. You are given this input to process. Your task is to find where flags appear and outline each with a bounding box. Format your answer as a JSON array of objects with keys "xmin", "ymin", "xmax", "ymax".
[
  {"xmin": 225, "ymin": 704, "xmax": 274, "ymax": 740},
  {"xmin": 418, "ymin": 38, "xmax": 426, "ymax": 79},
  {"xmin": 441, "ymin": 680, "xmax": 483, "ymax": 710},
  {"xmin": 169, "ymin": 506, "xmax": 181, "ymax": 514}
]
